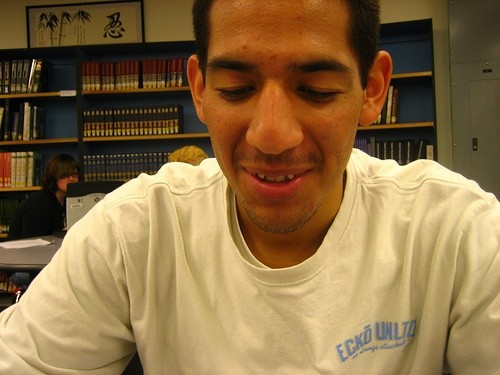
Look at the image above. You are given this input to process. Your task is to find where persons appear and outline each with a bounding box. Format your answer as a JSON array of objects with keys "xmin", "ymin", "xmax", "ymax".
[
  {"xmin": 0, "ymin": 0, "xmax": 500, "ymax": 375},
  {"xmin": 5, "ymin": 155, "xmax": 81, "ymax": 288},
  {"xmin": 169, "ymin": 146, "xmax": 208, "ymax": 166}
]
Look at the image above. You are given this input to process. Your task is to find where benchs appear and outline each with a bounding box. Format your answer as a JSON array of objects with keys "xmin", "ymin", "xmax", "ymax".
[{"xmin": 0, "ymin": 91, "xmax": 78, "ymax": 239}]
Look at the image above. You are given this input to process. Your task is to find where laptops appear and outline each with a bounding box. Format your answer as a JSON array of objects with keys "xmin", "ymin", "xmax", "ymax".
[{"xmin": 53, "ymin": 178, "xmax": 128, "ymax": 238}]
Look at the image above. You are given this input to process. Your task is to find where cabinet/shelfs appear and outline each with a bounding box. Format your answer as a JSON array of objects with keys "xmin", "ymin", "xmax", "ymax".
[{"xmin": 82, "ymin": 71, "xmax": 434, "ymax": 142}]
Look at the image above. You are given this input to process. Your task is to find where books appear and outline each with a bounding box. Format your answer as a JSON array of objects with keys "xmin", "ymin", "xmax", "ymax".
[
  {"xmin": 352, "ymin": 137, "xmax": 434, "ymax": 167},
  {"xmin": 371, "ymin": 87, "xmax": 399, "ymax": 126},
  {"xmin": 83, "ymin": 58, "xmax": 184, "ymax": 182},
  {"xmin": 0, "ymin": 58, "xmax": 46, "ymax": 292}
]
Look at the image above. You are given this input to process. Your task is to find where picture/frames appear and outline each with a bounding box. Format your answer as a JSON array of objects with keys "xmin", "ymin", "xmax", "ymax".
[{"xmin": 25, "ymin": 0, "xmax": 146, "ymax": 50}]
